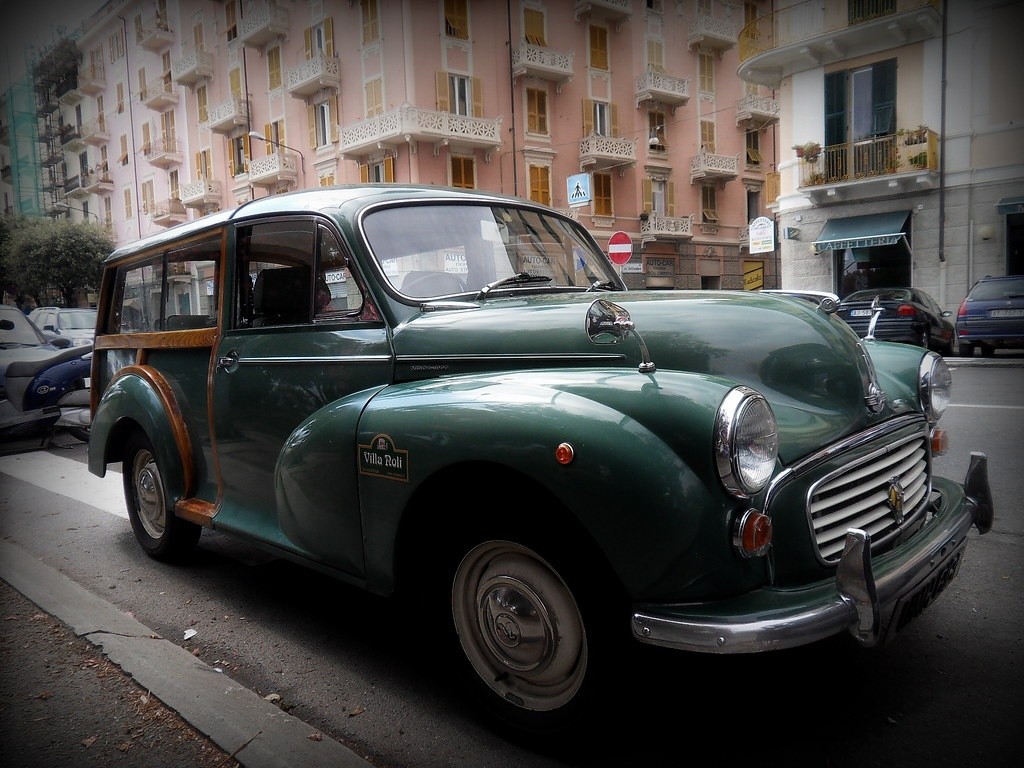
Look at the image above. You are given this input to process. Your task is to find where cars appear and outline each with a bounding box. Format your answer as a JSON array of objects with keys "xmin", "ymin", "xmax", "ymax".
[
  {"xmin": 0, "ymin": 306, "xmax": 131, "ymax": 346},
  {"xmin": 835, "ymin": 287, "xmax": 954, "ymax": 356},
  {"xmin": 87, "ymin": 183, "xmax": 995, "ymax": 752},
  {"xmin": 955, "ymin": 274, "xmax": 1024, "ymax": 356}
]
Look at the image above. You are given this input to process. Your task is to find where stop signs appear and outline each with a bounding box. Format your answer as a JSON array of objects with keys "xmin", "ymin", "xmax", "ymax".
[{"xmin": 607, "ymin": 231, "xmax": 633, "ymax": 266}]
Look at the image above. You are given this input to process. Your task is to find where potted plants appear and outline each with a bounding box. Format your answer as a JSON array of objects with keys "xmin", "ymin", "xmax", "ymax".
[
  {"xmin": 792, "ymin": 144, "xmax": 804, "ymax": 156},
  {"xmin": 898, "ymin": 124, "xmax": 929, "ymax": 146},
  {"xmin": 802, "ymin": 142, "xmax": 821, "ymax": 162},
  {"xmin": 234, "ymin": 162, "xmax": 244, "ymax": 174},
  {"xmin": 650, "ymin": 131, "xmax": 659, "ymax": 144}
]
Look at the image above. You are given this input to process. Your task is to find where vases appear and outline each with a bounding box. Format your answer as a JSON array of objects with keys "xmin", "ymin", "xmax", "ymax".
[{"xmin": 884, "ymin": 167, "xmax": 897, "ymax": 174}]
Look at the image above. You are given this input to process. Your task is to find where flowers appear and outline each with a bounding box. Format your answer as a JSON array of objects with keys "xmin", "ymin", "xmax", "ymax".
[{"xmin": 883, "ymin": 147, "xmax": 903, "ymax": 167}]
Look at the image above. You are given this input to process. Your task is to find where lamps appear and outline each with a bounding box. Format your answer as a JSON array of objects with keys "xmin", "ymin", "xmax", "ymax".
[
  {"xmin": 639, "ymin": 209, "xmax": 649, "ymax": 221},
  {"xmin": 979, "ymin": 225, "xmax": 993, "ymax": 240},
  {"xmin": 811, "ymin": 245, "xmax": 823, "ymax": 255}
]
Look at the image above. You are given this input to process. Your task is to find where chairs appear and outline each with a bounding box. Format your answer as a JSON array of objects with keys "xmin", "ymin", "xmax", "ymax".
[{"xmin": 253, "ymin": 267, "xmax": 320, "ymax": 328}]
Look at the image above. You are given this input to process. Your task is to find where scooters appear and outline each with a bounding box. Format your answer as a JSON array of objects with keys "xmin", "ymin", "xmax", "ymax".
[{"xmin": 0, "ymin": 345, "xmax": 94, "ymax": 443}]
